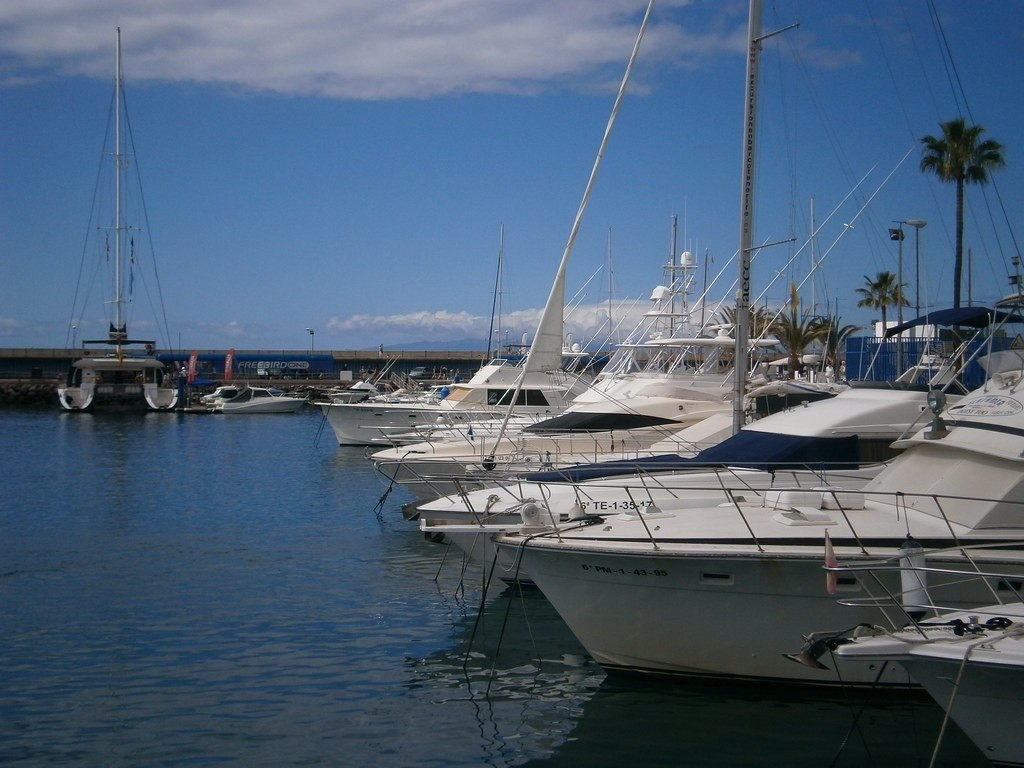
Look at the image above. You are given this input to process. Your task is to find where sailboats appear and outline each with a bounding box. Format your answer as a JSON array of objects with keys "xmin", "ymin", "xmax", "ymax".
[
  {"xmin": 54, "ymin": 27, "xmax": 184, "ymax": 414},
  {"xmin": 196, "ymin": 1, "xmax": 1024, "ymax": 768}
]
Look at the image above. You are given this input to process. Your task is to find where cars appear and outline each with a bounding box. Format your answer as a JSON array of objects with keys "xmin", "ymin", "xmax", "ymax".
[{"xmin": 408, "ymin": 367, "xmax": 433, "ymax": 380}]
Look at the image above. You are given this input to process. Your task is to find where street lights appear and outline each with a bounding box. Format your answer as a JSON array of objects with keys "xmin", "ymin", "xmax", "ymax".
[
  {"xmin": 307, "ymin": 328, "xmax": 315, "ymax": 354},
  {"xmin": 72, "ymin": 325, "xmax": 77, "ymax": 350}
]
